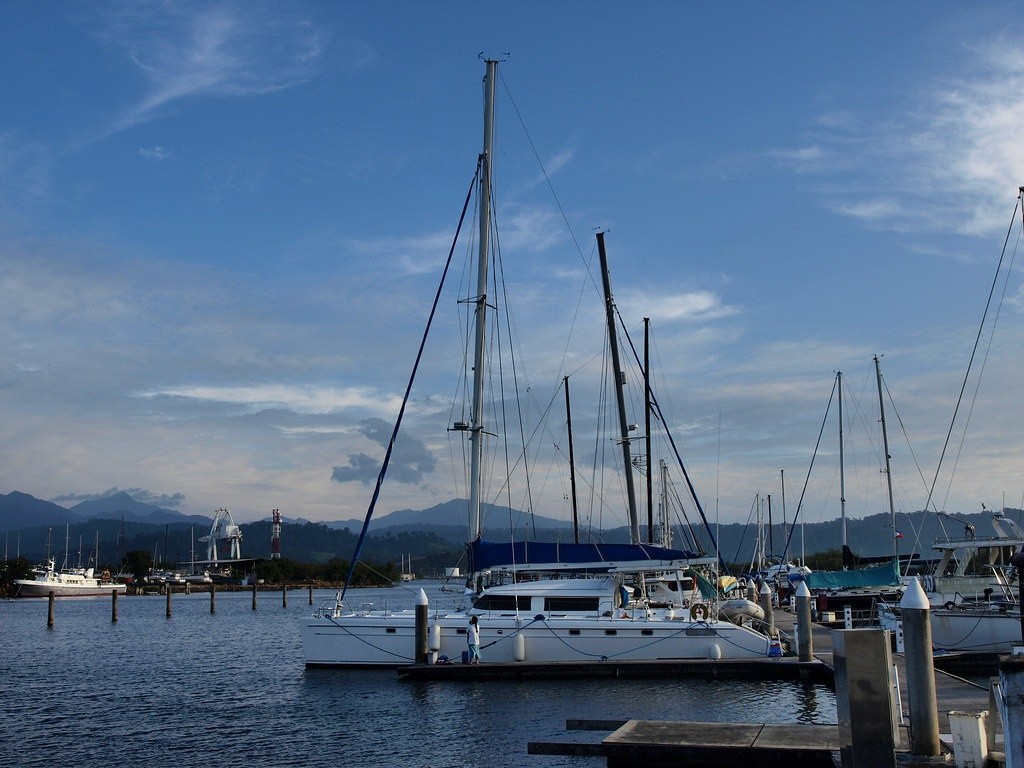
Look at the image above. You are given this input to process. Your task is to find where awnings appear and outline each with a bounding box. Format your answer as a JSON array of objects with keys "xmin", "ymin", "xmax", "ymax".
[{"xmin": 803, "ymin": 564, "xmax": 901, "ymax": 589}]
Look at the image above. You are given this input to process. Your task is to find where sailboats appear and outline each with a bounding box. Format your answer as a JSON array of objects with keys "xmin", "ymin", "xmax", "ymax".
[
  {"xmin": 7, "ymin": 513, "xmax": 213, "ymax": 599},
  {"xmin": 300, "ymin": 47, "xmax": 1023, "ymax": 669}
]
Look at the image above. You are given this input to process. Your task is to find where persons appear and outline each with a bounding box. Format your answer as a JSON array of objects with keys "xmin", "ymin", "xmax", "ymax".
[
  {"xmin": 697, "ymin": 569, "xmax": 710, "ymax": 579},
  {"xmin": 466, "ymin": 615, "xmax": 480, "ymax": 665}
]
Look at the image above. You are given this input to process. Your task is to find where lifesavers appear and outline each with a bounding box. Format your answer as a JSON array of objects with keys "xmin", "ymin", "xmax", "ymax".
[
  {"xmin": 691, "ymin": 603, "xmax": 708, "ymax": 620},
  {"xmin": 102, "ymin": 571, "xmax": 110, "ymax": 581}
]
